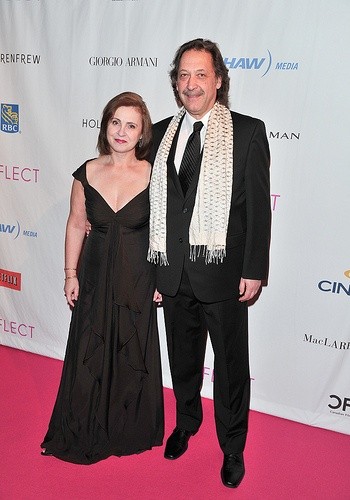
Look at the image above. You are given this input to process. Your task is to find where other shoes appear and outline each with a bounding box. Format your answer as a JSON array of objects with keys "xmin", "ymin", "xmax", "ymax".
[{"xmin": 41, "ymin": 448, "xmax": 54, "ymax": 455}]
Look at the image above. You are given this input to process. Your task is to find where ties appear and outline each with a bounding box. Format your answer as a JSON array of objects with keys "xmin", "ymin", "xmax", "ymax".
[{"xmin": 178, "ymin": 122, "xmax": 204, "ymax": 200}]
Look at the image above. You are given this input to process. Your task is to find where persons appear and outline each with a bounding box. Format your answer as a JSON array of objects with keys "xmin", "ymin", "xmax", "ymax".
[
  {"xmin": 40, "ymin": 91, "xmax": 153, "ymax": 457},
  {"xmin": 83, "ymin": 38, "xmax": 271, "ymax": 488}
]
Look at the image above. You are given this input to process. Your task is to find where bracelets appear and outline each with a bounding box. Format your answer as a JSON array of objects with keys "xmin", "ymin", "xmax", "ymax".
[
  {"xmin": 65, "ymin": 275, "xmax": 77, "ymax": 280},
  {"xmin": 64, "ymin": 267, "xmax": 77, "ymax": 270}
]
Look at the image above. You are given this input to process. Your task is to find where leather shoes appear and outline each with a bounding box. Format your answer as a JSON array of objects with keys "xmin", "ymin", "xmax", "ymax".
[
  {"xmin": 220, "ymin": 451, "xmax": 246, "ymax": 487},
  {"xmin": 164, "ymin": 425, "xmax": 200, "ymax": 460}
]
